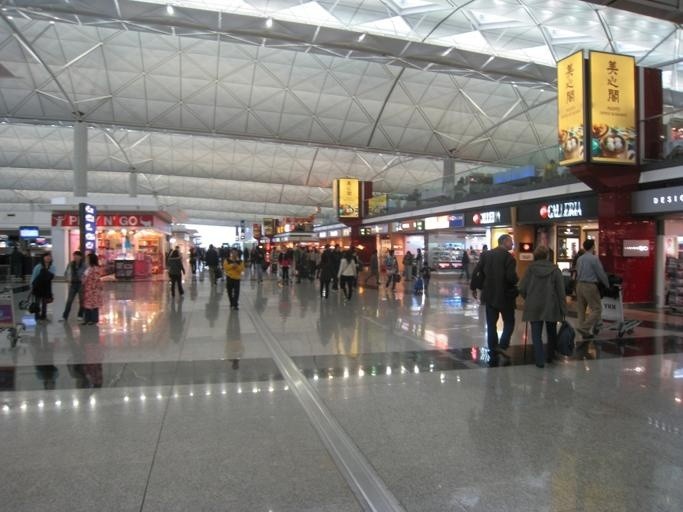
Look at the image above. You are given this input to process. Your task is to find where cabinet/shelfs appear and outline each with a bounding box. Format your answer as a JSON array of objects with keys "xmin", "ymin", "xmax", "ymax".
[{"xmin": 96, "ymin": 238, "xmax": 160, "ymax": 280}]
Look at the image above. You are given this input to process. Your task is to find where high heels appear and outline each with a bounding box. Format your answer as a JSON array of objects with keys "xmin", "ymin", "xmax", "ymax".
[
  {"xmin": 80, "ymin": 320, "xmax": 90, "ymax": 325},
  {"xmin": 88, "ymin": 321, "xmax": 99, "ymax": 326}
]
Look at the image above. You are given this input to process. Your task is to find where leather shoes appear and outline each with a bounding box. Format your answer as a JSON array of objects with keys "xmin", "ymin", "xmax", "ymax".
[
  {"xmin": 495, "ymin": 345, "xmax": 509, "ymax": 357},
  {"xmin": 489, "ymin": 358, "xmax": 500, "ymax": 368},
  {"xmin": 578, "ymin": 327, "xmax": 594, "ymax": 337}
]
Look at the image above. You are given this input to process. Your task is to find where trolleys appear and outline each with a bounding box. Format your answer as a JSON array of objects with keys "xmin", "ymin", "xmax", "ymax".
[
  {"xmin": 0, "ymin": 289, "xmax": 25, "ymax": 347},
  {"xmin": 591, "ymin": 282, "xmax": 643, "ymax": 337}
]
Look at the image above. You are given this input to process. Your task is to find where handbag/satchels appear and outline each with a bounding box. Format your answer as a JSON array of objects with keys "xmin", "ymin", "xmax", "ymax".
[
  {"xmin": 472, "ymin": 265, "xmax": 485, "ymax": 288},
  {"xmin": 19, "ymin": 299, "xmax": 41, "ymax": 313},
  {"xmin": 396, "ymin": 274, "xmax": 401, "ymax": 282}
]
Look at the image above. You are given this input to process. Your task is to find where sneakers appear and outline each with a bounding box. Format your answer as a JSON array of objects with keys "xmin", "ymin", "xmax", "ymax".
[
  {"xmin": 58, "ymin": 316, "xmax": 65, "ymax": 322},
  {"xmin": 76, "ymin": 316, "xmax": 83, "ymax": 321}
]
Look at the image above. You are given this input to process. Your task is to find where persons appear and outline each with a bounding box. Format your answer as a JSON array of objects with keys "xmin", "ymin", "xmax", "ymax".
[
  {"xmin": 79, "ymin": 254, "xmax": 103, "ymax": 327},
  {"xmin": 469, "ymin": 366, "xmax": 521, "ymax": 481},
  {"xmin": 454, "ymin": 175, "xmax": 467, "ymax": 202},
  {"xmin": 168, "ymin": 271, "xmax": 431, "ymax": 349},
  {"xmin": 78, "ymin": 324, "xmax": 106, "ymax": 389},
  {"xmin": 469, "ymin": 233, "xmax": 519, "ymax": 358},
  {"xmin": 225, "ymin": 308, "xmax": 244, "ymax": 371},
  {"xmin": 10, "ymin": 245, "xmax": 25, "ymax": 277},
  {"xmin": 58, "ymin": 319, "xmax": 90, "ymax": 392},
  {"xmin": 575, "ymin": 352, "xmax": 616, "ymax": 500},
  {"xmin": 543, "ymin": 159, "xmax": 557, "ymax": 182},
  {"xmin": 574, "ymin": 238, "xmax": 610, "ymax": 339},
  {"xmin": 163, "ymin": 241, "xmax": 487, "ymax": 312},
  {"xmin": 27, "ymin": 249, "xmax": 55, "ymax": 322},
  {"xmin": 519, "ymin": 369, "xmax": 573, "ymax": 479},
  {"xmin": 517, "ymin": 245, "xmax": 570, "ymax": 369},
  {"xmin": 411, "ymin": 186, "xmax": 422, "ymax": 208},
  {"xmin": 57, "ymin": 251, "xmax": 88, "ymax": 322},
  {"xmin": 28, "ymin": 323, "xmax": 60, "ymax": 394}
]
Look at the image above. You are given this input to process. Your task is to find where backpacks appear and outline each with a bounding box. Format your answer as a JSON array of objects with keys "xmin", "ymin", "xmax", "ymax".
[
  {"xmin": 561, "ymin": 267, "xmax": 578, "ymax": 296},
  {"xmin": 170, "ymin": 263, "xmax": 182, "ymax": 279},
  {"xmin": 557, "ymin": 324, "xmax": 577, "ymax": 355}
]
